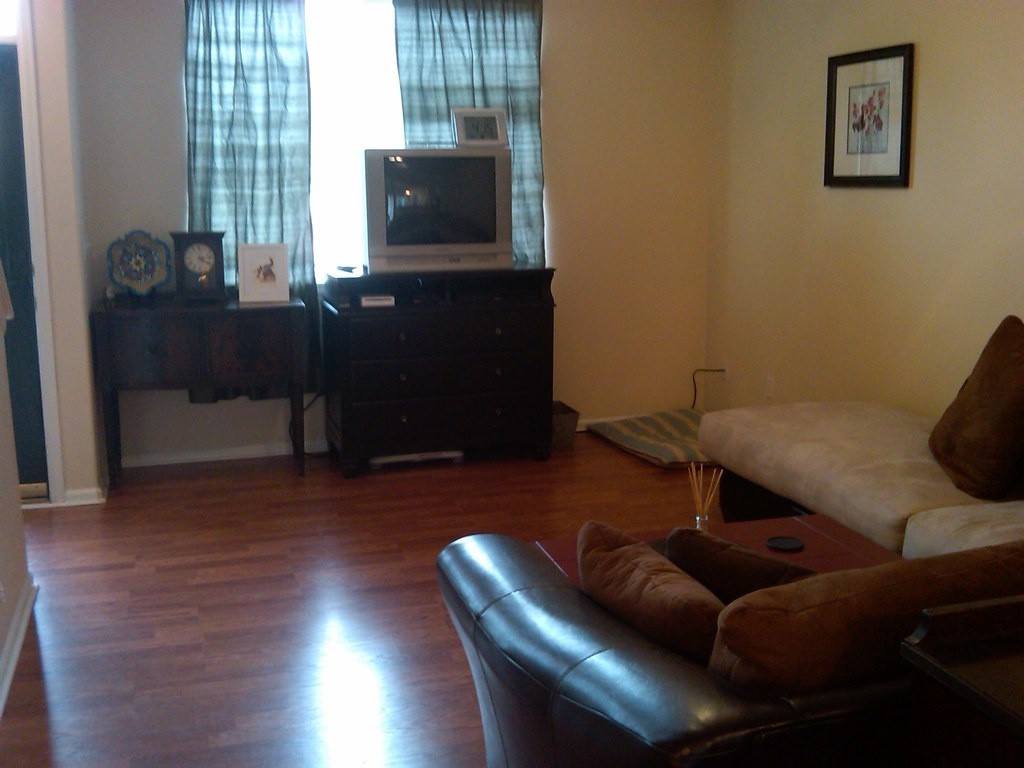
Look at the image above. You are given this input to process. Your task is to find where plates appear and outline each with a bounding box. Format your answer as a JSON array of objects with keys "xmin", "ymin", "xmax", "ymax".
[{"xmin": 107, "ymin": 229, "xmax": 171, "ymax": 295}]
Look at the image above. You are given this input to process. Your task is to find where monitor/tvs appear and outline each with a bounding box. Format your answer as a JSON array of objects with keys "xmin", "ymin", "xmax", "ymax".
[{"xmin": 364, "ymin": 149, "xmax": 513, "ymax": 271}]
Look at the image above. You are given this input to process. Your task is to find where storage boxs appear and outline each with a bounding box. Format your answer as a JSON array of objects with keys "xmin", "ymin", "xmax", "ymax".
[{"xmin": 552, "ymin": 400, "xmax": 580, "ymax": 446}]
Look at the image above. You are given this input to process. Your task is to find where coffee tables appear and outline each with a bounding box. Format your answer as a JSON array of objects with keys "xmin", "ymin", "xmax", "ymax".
[{"xmin": 710, "ymin": 515, "xmax": 904, "ymax": 573}]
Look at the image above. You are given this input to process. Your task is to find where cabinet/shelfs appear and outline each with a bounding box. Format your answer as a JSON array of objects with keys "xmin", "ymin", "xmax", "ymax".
[{"xmin": 321, "ymin": 267, "xmax": 556, "ymax": 475}]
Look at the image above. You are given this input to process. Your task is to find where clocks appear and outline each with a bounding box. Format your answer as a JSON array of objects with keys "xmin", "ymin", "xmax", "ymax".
[{"xmin": 171, "ymin": 232, "xmax": 225, "ymax": 299}]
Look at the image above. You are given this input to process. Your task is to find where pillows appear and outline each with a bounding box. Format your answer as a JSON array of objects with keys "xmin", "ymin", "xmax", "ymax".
[
  {"xmin": 928, "ymin": 315, "xmax": 1024, "ymax": 501},
  {"xmin": 587, "ymin": 409, "xmax": 718, "ymax": 468},
  {"xmin": 577, "ymin": 519, "xmax": 1024, "ymax": 700}
]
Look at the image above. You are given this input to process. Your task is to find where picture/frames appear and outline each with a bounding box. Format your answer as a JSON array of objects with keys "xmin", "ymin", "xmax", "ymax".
[
  {"xmin": 237, "ymin": 244, "xmax": 289, "ymax": 303},
  {"xmin": 824, "ymin": 43, "xmax": 914, "ymax": 187}
]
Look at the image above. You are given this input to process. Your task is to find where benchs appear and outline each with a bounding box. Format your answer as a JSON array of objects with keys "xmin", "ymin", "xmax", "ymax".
[{"xmin": 698, "ymin": 402, "xmax": 1024, "ymax": 559}]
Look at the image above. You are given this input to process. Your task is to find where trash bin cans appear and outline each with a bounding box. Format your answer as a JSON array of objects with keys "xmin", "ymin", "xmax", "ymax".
[{"xmin": 551, "ymin": 401, "xmax": 580, "ymax": 451}]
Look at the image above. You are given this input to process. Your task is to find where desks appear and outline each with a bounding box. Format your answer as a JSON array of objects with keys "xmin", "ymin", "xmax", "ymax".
[
  {"xmin": 901, "ymin": 595, "xmax": 1024, "ymax": 768},
  {"xmin": 89, "ymin": 294, "xmax": 314, "ymax": 477}
]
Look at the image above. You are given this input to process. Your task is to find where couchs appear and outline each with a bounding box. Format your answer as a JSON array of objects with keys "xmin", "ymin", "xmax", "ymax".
[{"xmin": 436, "ymin": 533, "xmax": 912, "ymax": 768}]
